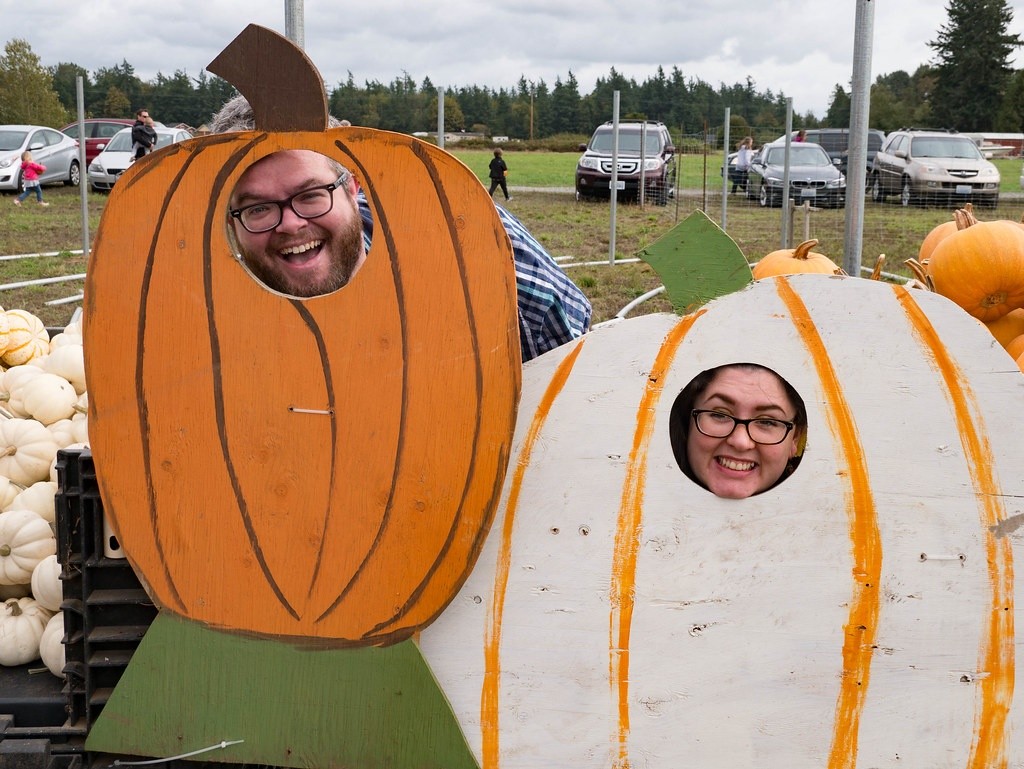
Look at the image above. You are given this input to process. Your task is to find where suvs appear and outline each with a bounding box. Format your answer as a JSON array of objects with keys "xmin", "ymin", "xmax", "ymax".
[
  {"xmin": 575, "ymin": 119, "xmax": 677, "ymax": 207},
  {"xmin": 870, "ymin": 126, "xmax": 1001, "ymax": 210},
  {"xmin": 720, "ymin": 129, "xmax": 887, "ymax": 194}
]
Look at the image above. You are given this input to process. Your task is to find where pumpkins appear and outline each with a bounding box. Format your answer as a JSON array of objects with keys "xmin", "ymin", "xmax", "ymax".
[
  {"xmin": 0, "ymin": 295, "xmax": 114, "ymax": 680},
  {"xmin": 750, "ymin": 202, "xmax": 1024, "ymax": 375}
]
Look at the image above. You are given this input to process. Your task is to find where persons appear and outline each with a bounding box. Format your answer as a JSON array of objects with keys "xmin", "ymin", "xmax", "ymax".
[
  {"xmin": 793, "ymin": 130, "xmax": 807, "ymax": 142},
  {"xmin": 13, "ymin": 151, "xmax": 49, "ymax": 206},
  {"xmin": 731, "ymin": 136, "xmax": 759, "ymax": 195},
  {"xmin": 215, "ymin": 90, "xmax": 593, "ymax": 361},
  {"xmin": 488, "ymin": 148, "xmax": 513, "ymax": 201},
  {"xmin": 675, "ymin": 367, "xmax": 807, "ymax": 500},
  {"xmin": 129, "ymin": 109, "xmax": 157, "ymax": 163}
]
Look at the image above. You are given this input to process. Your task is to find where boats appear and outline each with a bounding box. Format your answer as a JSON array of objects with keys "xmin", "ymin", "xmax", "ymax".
[{"xmin": 980, "ymin": 146, "xmax": 1016, "ymax": 157}]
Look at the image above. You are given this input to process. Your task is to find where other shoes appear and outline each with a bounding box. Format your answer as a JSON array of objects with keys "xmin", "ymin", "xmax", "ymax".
[
  {"xmin": 38, "ymin": 201, "xmax": 49, "ymax": 206},
  {"xmin": 14, "ymin": 199, "xmax": 21, "ymax": 206},
  {"xmin": 505, "ymin": 195, "xmax": 513, "ymax": 201}
]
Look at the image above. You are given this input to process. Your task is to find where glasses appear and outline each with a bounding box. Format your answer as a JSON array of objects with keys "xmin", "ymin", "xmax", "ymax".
[
  {"xmin": 141, "ymin": 116, "xmax": 146, "ymax": 118},
  {"xmin": 692, "ymin": 408, "xmax": 800, "ymax": 444},
  {"xmin": 230, "ymin": 171, "xmax": 349, "ymax": 234}
]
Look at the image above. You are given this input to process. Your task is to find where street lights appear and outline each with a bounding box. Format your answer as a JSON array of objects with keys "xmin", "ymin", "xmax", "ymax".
[{"xmin": 531, "ymin": 92, "xmax": 538, "ymax": 140}]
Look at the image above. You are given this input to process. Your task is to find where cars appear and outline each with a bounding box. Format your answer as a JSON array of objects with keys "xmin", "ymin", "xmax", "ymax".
[
  {"xmin": 88, "ymin": 127, "xmax": 193, "ymax": 193},
  {"xmin": 0, "ymin": 125, "xmax": 79, "ymax": 194},
  {"xmin": 745, "ymin": 143, "xmax": 846, "ymax": 208},
  {"xmin": 53, "ymin": 119, "xmax": 165, "ymax": 165}
]
